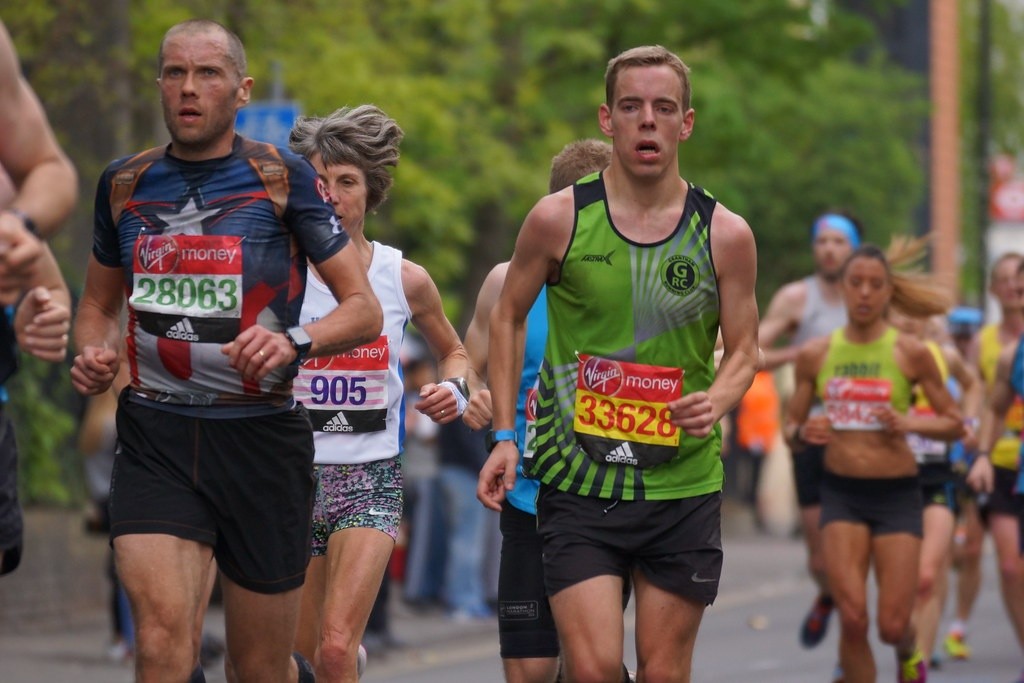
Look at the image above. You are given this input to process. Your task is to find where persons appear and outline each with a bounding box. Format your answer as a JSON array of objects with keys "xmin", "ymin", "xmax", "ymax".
[
  {"xmin": 0, "ymin": 0, "xmax": 630, "ymax": 683},
  {"xmin": 711, "ymin": 211, "xmax": 1024, "ymax": 683},
  {"xmin": 476, "ymin": 46, "xmax": 760, "ymax": 682}
]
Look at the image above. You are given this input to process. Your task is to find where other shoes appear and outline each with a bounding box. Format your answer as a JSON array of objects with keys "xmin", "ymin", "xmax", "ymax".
[
  {"xmin": 292, "ymin": 651, "xmax": 316, "ymax": 683},
  {"xmin": 358, "ymin": 645, "xmax": 367, "ymax": 679}
]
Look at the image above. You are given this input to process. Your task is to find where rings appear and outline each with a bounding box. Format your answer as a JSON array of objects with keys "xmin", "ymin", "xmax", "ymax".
[
  {"xmin": 259, "ymin": 350, "xmax": 268, "ymax": 361},
  {"xmin": 441, "ymin": 411, "xmax": 445, "ymax": 417}
]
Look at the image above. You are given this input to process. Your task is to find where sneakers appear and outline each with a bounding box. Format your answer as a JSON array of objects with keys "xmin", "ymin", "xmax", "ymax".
[
  {"xmin": 946, "ymin": 632, "xmax": 969, "ymax": 658},
  {"xmin": 896, "ymin": 641, "xmax": 926, "ymax": 683},
  {"xmin": 800, "ymin": 593, "xmax": 833, "ymax": 647}
]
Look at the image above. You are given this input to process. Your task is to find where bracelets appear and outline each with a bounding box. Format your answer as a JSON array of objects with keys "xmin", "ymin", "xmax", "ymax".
[
  {"xmin": 978, "ymin": 451, "xmax": 990, "ymax": 456},
  {"xmin": 6, "ymin": 206, "xmax": 38, "ymax": 235},
  {"xmin": 758, "ymin": 348, "xmax": 766, "ymax": 372}
]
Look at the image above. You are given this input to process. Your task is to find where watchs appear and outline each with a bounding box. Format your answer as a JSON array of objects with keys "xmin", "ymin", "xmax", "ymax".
[
  {"xmin": 282, "ymin": 326, "xmax": 312, "ymax": 363},
  {"xmin": 441, "ymin": 377, "xmax": 470, "ymax": 401},
  {"xmin": 965, "ymin": 417, "xmax": 980, "ymax": 430},
  {"xmin": 485, "ymin": 429, "xmax": 519, "ymax": 453}
]
